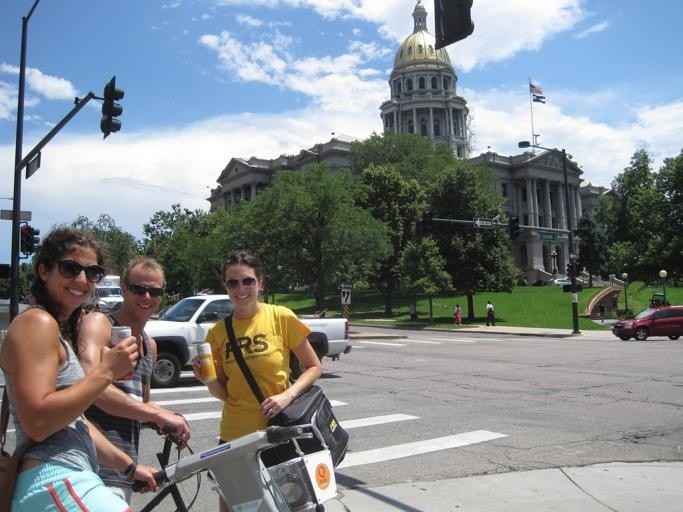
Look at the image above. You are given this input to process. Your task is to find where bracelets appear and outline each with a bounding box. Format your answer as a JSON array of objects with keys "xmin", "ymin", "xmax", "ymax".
[{"xmin": 119, "ymin": 458, "xmax": 139, "ymax": 481}]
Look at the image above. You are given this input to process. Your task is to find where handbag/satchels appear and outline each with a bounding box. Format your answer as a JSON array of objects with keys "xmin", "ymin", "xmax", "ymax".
[
  {"xmin": 267, "ymin": 386, "xmax": 349, "ymax": 467},
  {"xmin": 0, "ymin": 451, "xmax": 19, "ymax": 512}
]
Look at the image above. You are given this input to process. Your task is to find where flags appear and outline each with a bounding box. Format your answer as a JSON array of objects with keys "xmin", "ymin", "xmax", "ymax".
[
  {"xmin": 529, "ymin": 83, "xmax": 544, "ymax": 94},
  {"xmin": 532, "ymin": 95, "xmax": 546, "ymax": 105}
]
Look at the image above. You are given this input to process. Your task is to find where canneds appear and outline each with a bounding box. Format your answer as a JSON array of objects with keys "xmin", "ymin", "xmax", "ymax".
[
  {"xmin": 111, "ymin": 326, "xmax": 133, "ymax": 383},
  {"xmin": 197, "ymin": 342, "xmax": 218, "ymax": 383}
]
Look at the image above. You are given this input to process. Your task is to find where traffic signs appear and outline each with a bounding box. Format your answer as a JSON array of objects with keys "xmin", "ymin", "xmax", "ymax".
[
  {"xmin": 538, "ymin": 231, "xmax": 558, "ymax": 241},
  {"xmin": 472, "ymin": 218, "xmax": 492, "ymax": 229}
]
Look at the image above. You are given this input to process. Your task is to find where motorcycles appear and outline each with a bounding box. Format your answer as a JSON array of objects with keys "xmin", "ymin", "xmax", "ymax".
[{"xmin": 130, "ymin": 423, "xmax": 351, "ymax": 511}]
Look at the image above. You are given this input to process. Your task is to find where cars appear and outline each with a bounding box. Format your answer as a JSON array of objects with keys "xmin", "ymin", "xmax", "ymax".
[
  {"xmin": 648, "ymin": 292, "xmax": 670, "ymax": 308},
  {"xmin": 611, "ymin": 305, "xmax": 683, "ymax": 340},
  {"xmin": 18, "ymin": 294, "xmax": 30, "ymax": 304},
  {"xmin": 548, "ymin": 278, "xmax": 571, "ymax": 286},
  {"xmin": 531, "ymin": 280, "xmax": 548, "ymax": 285}
]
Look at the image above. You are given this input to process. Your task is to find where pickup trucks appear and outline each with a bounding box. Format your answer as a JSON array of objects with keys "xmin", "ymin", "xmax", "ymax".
[{"xmin": 141, "ymin": 294, "xmax": 351, "ymax": 388}]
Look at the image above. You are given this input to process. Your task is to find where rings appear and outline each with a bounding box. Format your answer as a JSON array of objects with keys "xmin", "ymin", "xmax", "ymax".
[
  {"xmin": 269, "ymin": 407, "xmax": 273, "ymax": 413},
  {"xmin": 181, "ymin": 433, "xmax": 186, "ymax": 437}
]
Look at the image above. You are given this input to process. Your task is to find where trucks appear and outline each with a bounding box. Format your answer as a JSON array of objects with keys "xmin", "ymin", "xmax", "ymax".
[{"xmin": 83, "ymin": 275, "xmax": 123, "ymax": 314}]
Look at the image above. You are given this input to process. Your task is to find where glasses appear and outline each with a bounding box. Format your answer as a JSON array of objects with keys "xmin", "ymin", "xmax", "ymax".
[
  {"xmin": 226, "ymin": 278, "xmax": 255, "ymax": 289},
  {"xmin": 129, "ymin": 284, "xmax": 163, "ymax": 297},
  {"xmin": 56, "ymin": 260, "xmax": 105, "ymax": 283}
]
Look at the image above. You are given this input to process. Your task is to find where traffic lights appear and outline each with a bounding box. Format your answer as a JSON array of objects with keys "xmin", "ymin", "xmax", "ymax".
[
  {"xmin": 508, "ymin": 214, "xmax": 519, "ymax": 240},
  {"xmin": 422, "ymin": 209, "xmax": 433, "ymax": 235},
  {"xmin": 574, "ymin": 259, "xmax": 582, "ymax": 278},
  {"xmin": 101, "ymin": 77, "xmax": 124, "ymax": 140},
  {"xmin": 20, "ymin": 225, "xmax": 31, "ymax": 255},
  {"xmin": 566, "ymin": 260, "xmax": 573, "ymax": 278},
  {"xmin": 31, "ymin": 228, "xmax": 40, "ymax": 253}
]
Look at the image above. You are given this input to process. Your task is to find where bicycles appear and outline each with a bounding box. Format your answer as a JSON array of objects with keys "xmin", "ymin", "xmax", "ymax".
[{"xmin": 139, "ymin": 411, "xmax": 190, "ymax": 511}]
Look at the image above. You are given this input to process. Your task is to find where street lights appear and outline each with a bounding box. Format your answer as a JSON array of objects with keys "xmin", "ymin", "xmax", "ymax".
[
  {"xmin": 518, "ymin": 141, "xmax": 581, "ymax": 334},
  {"xmin": 659, "ymin": 270, "xmax": 667, "ymax": 305},
  {"xmin": 550, "ymin": 251, "xmax": 557, "ymax": 274},
  {"xmin": 0, "ymin": 196, "xmax": 13, "ymax": 201},
  {"xmin": 622, "ymin": 273, "xmax": 627, "ymax": 313}
]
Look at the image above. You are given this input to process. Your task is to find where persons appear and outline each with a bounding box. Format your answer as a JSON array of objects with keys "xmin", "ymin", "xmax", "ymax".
[
  {"xmin": 190, "ymin": 250, "xmax": 323, "ymax": 511},
  {"xmin": 598, "ymin": 301, "xmax": 607, "ymax": 323},
  {"xmin": 649, "ymin": 299, "xmax": 671, "ymax": 308},
  {"xmin": 485, "ymin": 300, "xmax": 495, "ymax": 326},
  {"xmin": 0, "ymin": 225, "xmax": 190, "ymax": 511},
  {"xmin": 75, "ymin": 256, "xmax": 190, "ymax": 505},
  {"xmin": 409, "ymin": 300, "xmax": 416, "ymax": 320},
  {"xmin": 453, "ymin": 304, "xmax": 462, "ymax": 324}
]
